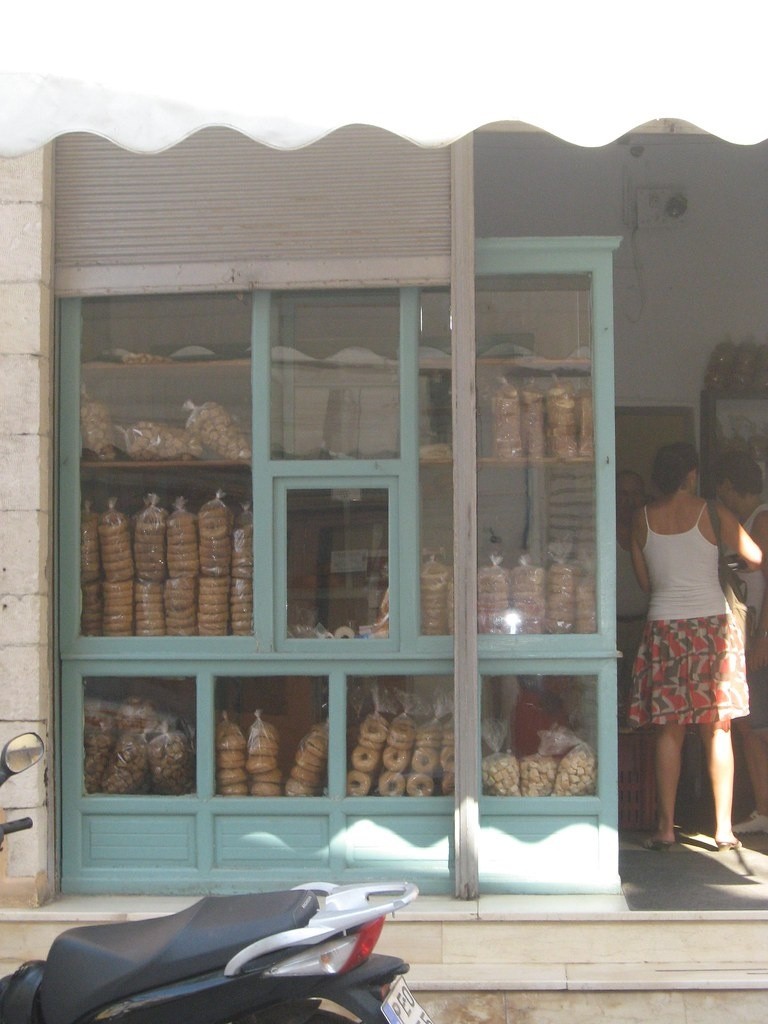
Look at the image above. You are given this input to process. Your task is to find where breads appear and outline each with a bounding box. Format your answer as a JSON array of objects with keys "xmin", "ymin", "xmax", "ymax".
[{"xmin": 80, "ymin": 387, "xmax": 599, "ymax": 797}]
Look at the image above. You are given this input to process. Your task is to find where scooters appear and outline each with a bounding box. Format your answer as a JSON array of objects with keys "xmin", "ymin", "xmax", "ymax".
[{"xmin": 1, "ymin": 729, "xmax": 436, "ymax": 1024}]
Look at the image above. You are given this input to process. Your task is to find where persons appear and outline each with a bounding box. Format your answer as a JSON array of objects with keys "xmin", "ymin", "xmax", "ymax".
[{"xmin": 616, "ymin": 442, "xmax": 768, "ymax": 851}]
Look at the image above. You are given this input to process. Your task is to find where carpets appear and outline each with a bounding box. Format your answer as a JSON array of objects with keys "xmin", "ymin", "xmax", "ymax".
[{"xmin": 619, "ymin": 850, "xmax": 768, "ymax": 911}]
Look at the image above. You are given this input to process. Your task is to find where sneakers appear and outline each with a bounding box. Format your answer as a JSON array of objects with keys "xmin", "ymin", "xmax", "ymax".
[{"xmin": 733, "ymin": 810, "xmax": 768, "ymax": 836}]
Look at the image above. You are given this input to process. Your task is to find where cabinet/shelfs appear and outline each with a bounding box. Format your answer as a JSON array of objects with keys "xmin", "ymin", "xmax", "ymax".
[{"xmin": 52, "ymin": 235, "xmax": 623, "ymax": 895}]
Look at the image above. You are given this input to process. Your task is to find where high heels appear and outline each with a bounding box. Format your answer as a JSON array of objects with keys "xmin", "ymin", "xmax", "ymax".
[
  {"xmin": 717, "ymin": 839, "xmax": 742, "ymax": 852},
  {"xmin": 644, "ymin": 836, "xmax": 675, "ymax": 849}
]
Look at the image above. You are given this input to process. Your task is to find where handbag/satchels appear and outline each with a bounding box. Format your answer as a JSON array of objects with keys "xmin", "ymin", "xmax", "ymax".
[{"xmin": 718, "ymin": 560, "xmax": 756, "ymax": 652}]
